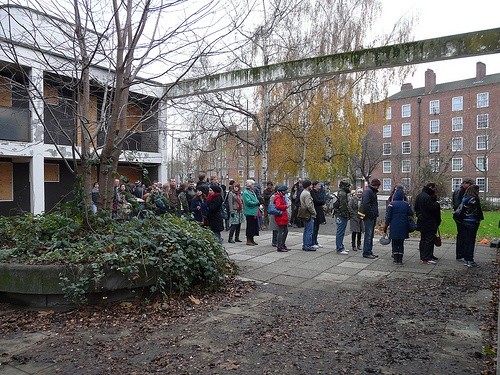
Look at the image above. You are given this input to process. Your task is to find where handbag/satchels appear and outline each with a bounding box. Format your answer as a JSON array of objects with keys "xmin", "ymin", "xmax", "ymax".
[
  {"xmin": 452, "ymin": 206, "xmax": 463, "ymax": 223},
  {"xmin": 357, "ymin": 210, "xmax": 367, "ymax": 220},
  {"xmin": 379, "ymin": 232, "xmax": 391, "ymax": 245},
  {"xmin": 268, "ymin": 204, "xmax": 283, "ymax": 215},
  {"xmin": 297, "ymin": 208, "xmax": 311, "ymax": 222},
  {"xmin": 407, "ymin": 216, "xmax": 418, "ymax": 233},
  {"xmin": 435, "ymin": 227, "xmax": 442, "ymax": 247},
  {"xmin": 219, "ymin": 204, "xmax": 228, "ymax": 220}
]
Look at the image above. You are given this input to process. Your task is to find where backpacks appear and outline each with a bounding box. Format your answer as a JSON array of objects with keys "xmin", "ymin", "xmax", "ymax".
[{"xmin": 330, "ymin": 189, "xmax": 343, "ymax": 210}]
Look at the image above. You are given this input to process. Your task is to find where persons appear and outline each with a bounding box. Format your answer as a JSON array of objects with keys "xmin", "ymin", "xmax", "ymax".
[{"xmin": 91, "ymin": 173, "xmax": 485, "ymax": 266}]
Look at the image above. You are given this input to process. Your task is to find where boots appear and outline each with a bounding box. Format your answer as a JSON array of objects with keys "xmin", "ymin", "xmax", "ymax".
[{"xmin": 392, "ymin": 253, "xmax": 403, "ymax": 264}]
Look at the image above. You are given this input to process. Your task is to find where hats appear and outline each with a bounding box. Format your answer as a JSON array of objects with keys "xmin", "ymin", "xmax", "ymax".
[
  {"xmin": 371, "ymin": 179, "xmax": 381, "ymax": 186},
  {"xmin": 463, "ymin": 177, "xmax": 476, "ymax": 184},
  {"xmin": 277, "ymin": 185, "xmax": 288, "ymax": 191},
  {"xmin": 302, "ymin": 180, "xmax": 312, "ymax": 188},
  {"xmin": 210, "ymin": 185, "xmax": 221, "ymax": 193}
]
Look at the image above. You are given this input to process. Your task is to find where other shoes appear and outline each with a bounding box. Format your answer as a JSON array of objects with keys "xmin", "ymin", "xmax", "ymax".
[
  {"xmin": 277, "ymin": 246, "xmax": 291, "ymax": 252},
  {"xmin": 463, "ymin": 260, "xmax": 477, "ymax": 267},
  {"xmin": 311, "ymin": 244, "xmax": 324, "ymax": 249},
  {"xmin": 228, "ymin": 239, "xmax": 235, "ymax": 243},
  {"xmin": 302, "ymin": 247, "xmax": 316, "ymax": 251},
  {"xmin": 235, "ymin": 238, "xmax": 243, "ymax": 242},
  {"xmin": 456, "ymin": 257, "xmax": 464, "ymax": 260},
  {"xmin": 363, "ymin": 254, "xmax": 379, "ymax": 259},
  {"xmin": 352, "ymin": 246, "xmax": 357, "ymax": 251},
  {"xmin": 338, "ymin": 249, "xmax": 349, "ymax": 255},
  {"xmin": 432, "ymin": 255, "xmax": 438, "ymax": 261},
  {"xmin": 357, "ymin": 246, "xmax": 362, "ymax": 250},
  {"xmin": 422, "ymin": 259, "xmax": 436, "ymax": 265},
  {"xmin": 245, "ymin": 240, "xmax": 258, "ymax": 246}
]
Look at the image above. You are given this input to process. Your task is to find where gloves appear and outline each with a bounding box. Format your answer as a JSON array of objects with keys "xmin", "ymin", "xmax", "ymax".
[{"xmin": 383, "ymin": 226, "xmax": 387, "ymax": 233}]
